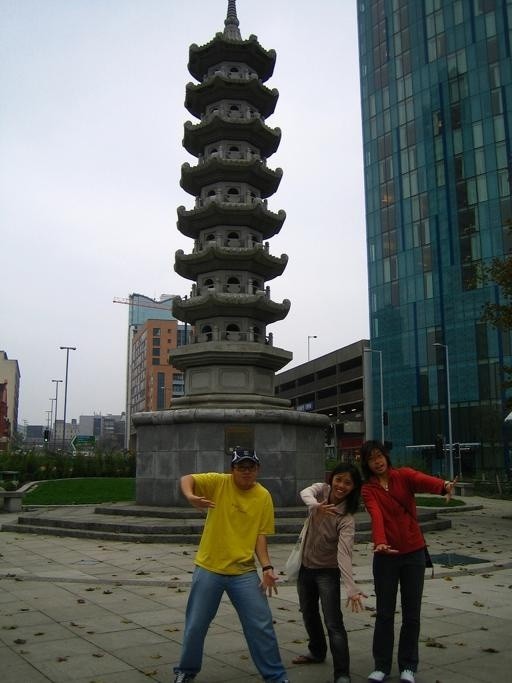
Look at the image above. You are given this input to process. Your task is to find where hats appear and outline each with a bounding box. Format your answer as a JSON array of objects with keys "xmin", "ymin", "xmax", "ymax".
[{"xmin": 232, "ymin": 447, "xmax": 259, "ymax": 463}]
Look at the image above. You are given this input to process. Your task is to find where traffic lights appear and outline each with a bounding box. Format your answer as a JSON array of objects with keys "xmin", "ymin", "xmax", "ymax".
[
  {"xmin": 435, "ymin": 433, "xmax": 444, "ymax": 459},
  {"xmin": 455, "ymin": 443, "xmax": 460, "ymax": 458},
  {"xmin": 325, "ymin": 424, "xmax": 331, "ymax": 445},
  {"xmin": 43, "ymin": 430, "xmax": 50, "ymax": 442}
]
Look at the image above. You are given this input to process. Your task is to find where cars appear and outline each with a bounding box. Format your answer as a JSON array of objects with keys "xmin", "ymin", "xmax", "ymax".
[{"xmin": 56, "ymin": 448, "xmax": 92, "ymax": 457}]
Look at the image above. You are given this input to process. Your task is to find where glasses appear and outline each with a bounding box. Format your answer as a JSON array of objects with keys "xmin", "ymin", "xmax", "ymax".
[{"xmin": 233, "ymin": 463, "xmax": 256, "ymax": 471}]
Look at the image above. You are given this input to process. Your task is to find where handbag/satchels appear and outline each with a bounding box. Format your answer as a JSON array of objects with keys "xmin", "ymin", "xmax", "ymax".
[{"xmin": 286, "ymin": 519, "xmax": 310, "ymax": 579}]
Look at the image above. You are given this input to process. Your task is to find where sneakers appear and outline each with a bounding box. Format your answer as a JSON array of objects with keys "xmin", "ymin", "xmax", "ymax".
[
  {"xmin": 367, "ymin": 671, "xmax": 387, "ymax": 682},
  {"xmin": 400, "ymin": 670, "xmax": 414, "ymax": 683},
  {"xmin": 293, "ymin": 655, "xmax": 322, "ymax": 664},
  {"xmin": 173, "ymin": 671, "xmax": 194, "ymax": 683}
]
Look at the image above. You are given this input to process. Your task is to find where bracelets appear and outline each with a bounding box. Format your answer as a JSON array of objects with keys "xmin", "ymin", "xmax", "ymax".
[{"xmin": 262, "ymin": 565, "xmax": 274, "ymax": 573}]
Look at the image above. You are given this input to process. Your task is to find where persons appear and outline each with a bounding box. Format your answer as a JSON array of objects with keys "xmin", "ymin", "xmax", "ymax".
[
  {"xmin": 291, "ymin": 461, "xmax": 368, "ymax": 682},
  {"xmin": 172, "ymin": 446, "xmax": 289, "ymax": 683},
  {"xmin": 360, "ymin": 439, "xmax": 461, "ymax": 683}
]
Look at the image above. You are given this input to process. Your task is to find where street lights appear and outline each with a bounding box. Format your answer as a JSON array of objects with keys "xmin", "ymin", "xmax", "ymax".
[
  {"xmin": 51, "ymin": 379, "xmax": 63, "ymax": 452},
  {"xmin": 433, "ymin": 342, "xmax": 455, "ymax": 496},
  {"xmin": 49, "ymin": 397, "xmax": 57, "ymax": 432},
  {"xmin": 60, "ymin": 345, "xmax": 78, "ymax": 452},
  {"xmin": 307, "ymin": 335, "xmax": 317, "ymax": 361},
  {"xmin": 45, "ymin": 410, "xmax": 52, "ymax": 430},
  {"xmin": 363, "ymin": 347, "xmax": 385, "ymax": 469}
]
